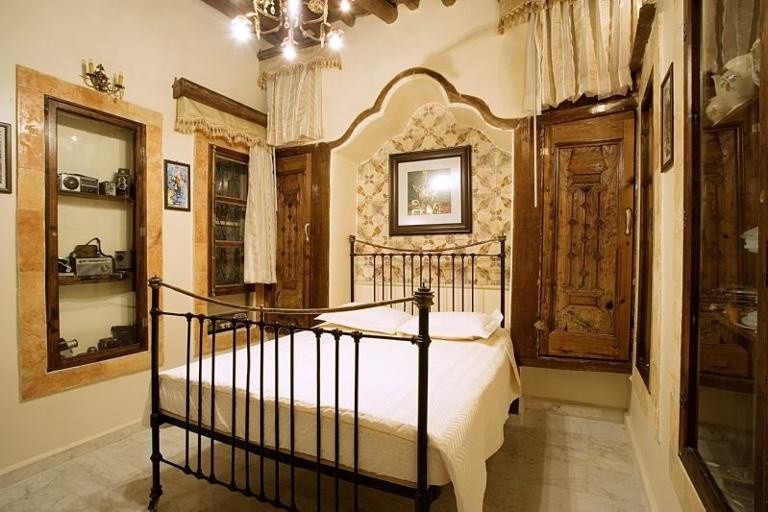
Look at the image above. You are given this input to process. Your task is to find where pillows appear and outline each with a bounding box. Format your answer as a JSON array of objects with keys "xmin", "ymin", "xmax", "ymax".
[{"xmin": 316, "ymin": 301, "xmax": 505, "ymax": 341}]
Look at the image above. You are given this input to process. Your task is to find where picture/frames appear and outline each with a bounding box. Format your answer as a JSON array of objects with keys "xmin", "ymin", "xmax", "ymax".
[
  {"xmin": 387, "ymin": 144, "xmax": 473, "ymax": 237},
  {"xmin": 162, "ymin": 158, "xmax": 190, "ymax": 212},
  {"xmin": 659, "ymin": 61, "xmax": 674, "ymax": 174},
  {"xmin": 0, "ymin": 121, "xmax": 14, "ymax": 195}
]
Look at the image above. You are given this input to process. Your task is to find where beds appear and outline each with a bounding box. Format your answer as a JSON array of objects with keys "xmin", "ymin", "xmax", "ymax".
[{"xmin": 147, "ymin": 235, "xmax": 512, "ymax": 510}]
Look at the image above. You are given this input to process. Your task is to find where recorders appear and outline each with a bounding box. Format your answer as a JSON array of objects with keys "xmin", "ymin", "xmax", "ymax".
[
  {"xmin": 57, "ymin": 172, "xmax": 100, "ymax": 196},
  {"xmin": 75, "ymin": 256, "xmax": 113, "ymax": 276}
]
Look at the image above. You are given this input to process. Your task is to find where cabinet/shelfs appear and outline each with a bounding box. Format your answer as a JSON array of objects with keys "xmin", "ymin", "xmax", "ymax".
[
  {"xmin": 678, "ymin": 0, "xmax": 767, "ymax": 511},
  {"xmin": 44, "ymin": 95, "xmax": 148, "ymax": 374}
]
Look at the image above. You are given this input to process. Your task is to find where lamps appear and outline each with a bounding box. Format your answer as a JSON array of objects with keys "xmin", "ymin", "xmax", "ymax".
[
  {"xmin": 231, "ymin": 0, "xmax": 351, "ymax": 60},
  {"xmin": 86, "ymin": 58, "xmax": 125, "ymax": 94}
]
